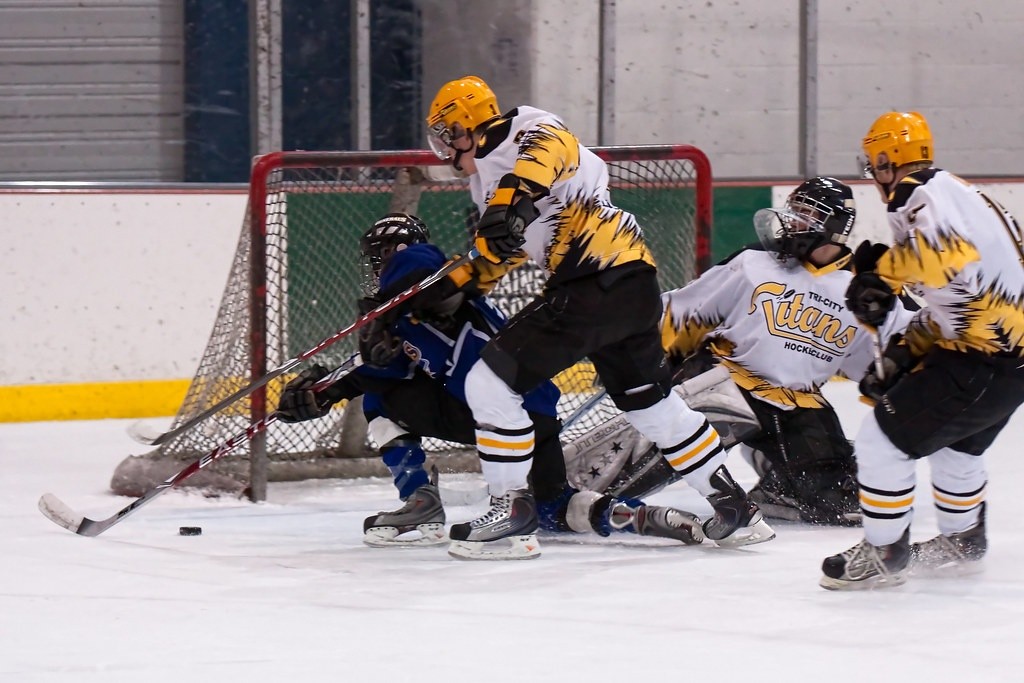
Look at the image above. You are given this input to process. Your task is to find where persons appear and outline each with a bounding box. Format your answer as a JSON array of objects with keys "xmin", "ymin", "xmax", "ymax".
[
  {"xmin": 426, "ymin": 72, "xmax": 776, "ymax": 563},
  {"xmin": 815, "ymin": 108, "xmax": 1024, "ymax": 586},
  {"xmin": 661, "ymin": 172, "xmax": 915, "ymax": 526},
  {"xmin": 277, "ymin": 214, "xmax": 702, "ymax": 542}
]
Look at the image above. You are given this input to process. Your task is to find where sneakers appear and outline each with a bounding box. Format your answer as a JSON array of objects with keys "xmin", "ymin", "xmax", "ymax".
[
  {"xmin": 905, "ymin": 501, "xmax": 988, "ymax": 578},
  {"xmin": 608, "ymin": 502, "xmax": 706, "ymax": 545},
  {"xmin": 364, "ymin": 464, "xmax": 449, "ymax": 547},
  {"xmin": 819, "ymin": 507, "xmax": 910, "ymax": 589},
  {"xmin": 448, "ymin": 489, "xmax": 541, "ymax": 560},
  {"xmin": 701, "ymin": 464, "xmax": 776, "ymax": 547}
]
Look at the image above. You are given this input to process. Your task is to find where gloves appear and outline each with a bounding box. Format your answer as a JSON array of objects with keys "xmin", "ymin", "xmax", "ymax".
[
  {"xmin": 845, "ymin": 274, "xmax": 896, "ymax": 327},
  {"xmin": 475, "ymin": 171, "xmax": 527, "ymax": 264},
  {"xmin": 859, "ymin": 331, "xmax": 921, "ymax": 400},
  {"xmin": 854, "ymin": 240, "xmax": 891, "ymax": 272},
  {"xmin": 276, "ymin": 364, "xmax": 343, "ymax": 424},
  {"xmin": 409, "ymin": 260, "xmax": 471, "ymax": 322},
  {"xmin": 357, "ymin": 297, "xmax": 403, "ymax": 370}
]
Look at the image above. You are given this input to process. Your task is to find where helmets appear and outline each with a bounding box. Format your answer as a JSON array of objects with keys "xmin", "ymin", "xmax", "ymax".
[
  {"xmin": 425, "ymin": 75, "xmax": 501, "ymax": 141},
  {"xmin": 862, "ymin": 109, "xmax": 934, "ymax": 168},
  {"xmin": 361, "ymin": 211, "xmax": 433, "ymax": 277},
  {"xmin": 789, "ymin": 176, "xmax": 857, "ymax": 244}
]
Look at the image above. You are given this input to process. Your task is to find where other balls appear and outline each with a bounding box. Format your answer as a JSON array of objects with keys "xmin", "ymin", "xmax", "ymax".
[{"xmin": 179, "ymin": 526, "xmax": 202, "ymax": 536}]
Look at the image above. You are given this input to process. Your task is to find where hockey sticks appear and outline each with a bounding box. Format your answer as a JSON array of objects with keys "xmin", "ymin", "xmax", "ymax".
[
  {"xmin": 869, "ymin": 325, "xmax": 897, "ymax": 414},
  {"xmin": 126, "ymin": 234, "xmax": 530, "ymax": 448},
  {"xmin": 559, "ymin": 386, "xmax": 610, "ymax": 434},
  {"xmin": 36, "ymin": 351, "xmax": 365, "ymax": 539}
]
